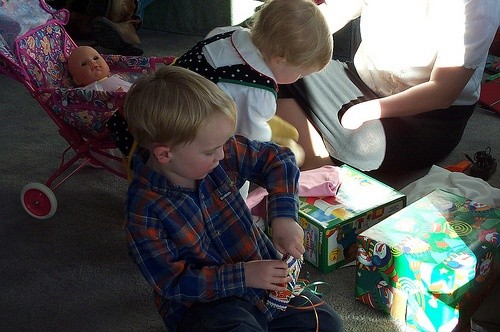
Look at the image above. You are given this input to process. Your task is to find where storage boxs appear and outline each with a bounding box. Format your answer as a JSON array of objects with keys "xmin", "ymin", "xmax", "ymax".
[
  {"xmin": 266, "ymin": 162, "xmax": 408, "ymax": 274},
  {"xmin": 356, "ymin": 187, "xmax": 500, "ymax": 332}
]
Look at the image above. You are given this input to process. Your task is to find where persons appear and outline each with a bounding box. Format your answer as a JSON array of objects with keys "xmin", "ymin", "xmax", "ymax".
[
  {"xmin": 106, "ymin": 0, "xmax": 334, "ymax": 205},
  {"xmin": 124, "ymin": 65, "xmax": 343, "ymax": 332},
  {"xmin": 275, "ymin": 0, "xmax": 500, "ymax": 177},
  {"xmin": 68, "ymin": 46, "xmax": 133, "ymax": 92}
]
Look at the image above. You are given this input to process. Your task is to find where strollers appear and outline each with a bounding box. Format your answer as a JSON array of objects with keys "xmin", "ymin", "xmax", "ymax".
[{"xmin": 1, "ymin": 1, "xmax": 178, "ymax": 219}]
